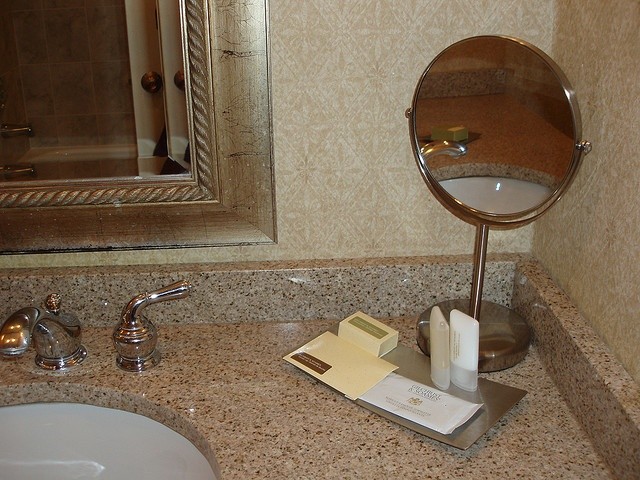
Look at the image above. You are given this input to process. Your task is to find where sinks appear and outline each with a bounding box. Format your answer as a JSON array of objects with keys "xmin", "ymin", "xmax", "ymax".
[{"xmin": 1, "ymin": 381, "xmax": 223, "ymax": 479}]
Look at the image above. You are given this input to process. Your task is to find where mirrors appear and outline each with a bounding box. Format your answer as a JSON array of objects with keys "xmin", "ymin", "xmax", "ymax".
[
  {"xmin": 0, "ymin": 3, "xmax": 279, "ymax": 254},
  {"xmin": 410, "ymin": 34, "xmax": 583, "ymax": 222}
]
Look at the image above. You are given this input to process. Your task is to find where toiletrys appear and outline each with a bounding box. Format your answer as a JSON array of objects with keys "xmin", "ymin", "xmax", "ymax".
[
  {"xmin": 449, "ymin": 308, "xmax": 479, "ymax": 393},
  {"xmin": 429, "ymin": 305, "xmax": 451, "ymax": 391}
]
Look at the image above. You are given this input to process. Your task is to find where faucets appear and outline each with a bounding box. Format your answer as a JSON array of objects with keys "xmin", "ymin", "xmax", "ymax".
[{"xmin": 1, "ymin": 293, "xmax": 86, "ymax": 372}]
